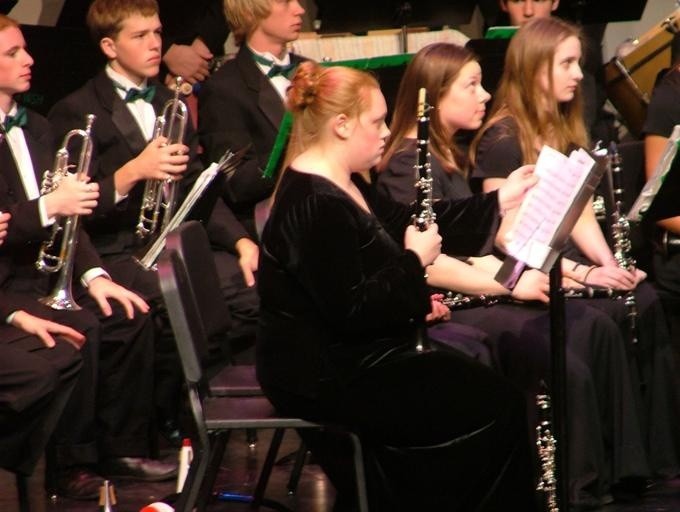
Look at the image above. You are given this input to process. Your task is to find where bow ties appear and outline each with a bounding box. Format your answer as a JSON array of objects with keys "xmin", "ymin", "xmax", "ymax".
[
  {"xmin": 252, "ymin": 52, "xmax": 299, "ymax": 81},
  {"xmin": 0, "ymin": 107, "xmax": 30, "ymax": 134},
  {"xmin": 111, "ymin": 79, "xmax": 158, "ymax": 105}
]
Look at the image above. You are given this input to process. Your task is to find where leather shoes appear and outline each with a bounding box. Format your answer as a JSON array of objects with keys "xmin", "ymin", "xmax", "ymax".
[
  {"xmin": 43, "ymin": 468, "xmax": 117, "ymax": 501},
  {"xmin": 103, "ymin": 455, "xmax": 179, "ymax": 485}
]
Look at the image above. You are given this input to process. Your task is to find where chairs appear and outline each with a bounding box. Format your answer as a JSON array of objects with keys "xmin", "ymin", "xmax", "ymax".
[
  {"xmin": 158, "ymin": 249, "xmax": 369, "ymax": 512},
  {"xmin": 167, "ymin": 220, "xmax": 310, "ymax": 492},
  {"xmin": 607, "ymin": 140, "xmax": 648, "ymax": 255}
]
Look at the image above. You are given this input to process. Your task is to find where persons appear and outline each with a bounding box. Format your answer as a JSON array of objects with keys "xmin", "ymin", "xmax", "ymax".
[
  {"xmin": 474, "ymin": 18, "xmax": 680, "ymax": 461},
  {"xmin": 643, "ymin": 62, "xmax": 680, "ymax": 236},
  {"xmin": 253, "ymin": 62, "xmax": 506, "ymax": 512},
  {"xmin": 0, "ymin": 0, "xmax": 309, "ymax": 500},
  {"xmin": 383, "ymin": 41, "xmax": 650, "ymax": 512},
  {"xmin": 500, "ymin": 0, "xmax": 559, "ymax": 26}
]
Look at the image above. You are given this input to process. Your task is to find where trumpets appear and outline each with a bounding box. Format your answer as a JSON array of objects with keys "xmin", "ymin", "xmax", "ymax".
[
  {"xmin": 35, "ymin": 113, "xmax": 97, "ymax": 312},
  {"xmin": 133, "ymin": 76, "xmax": 188, "ymax": 272}
]
